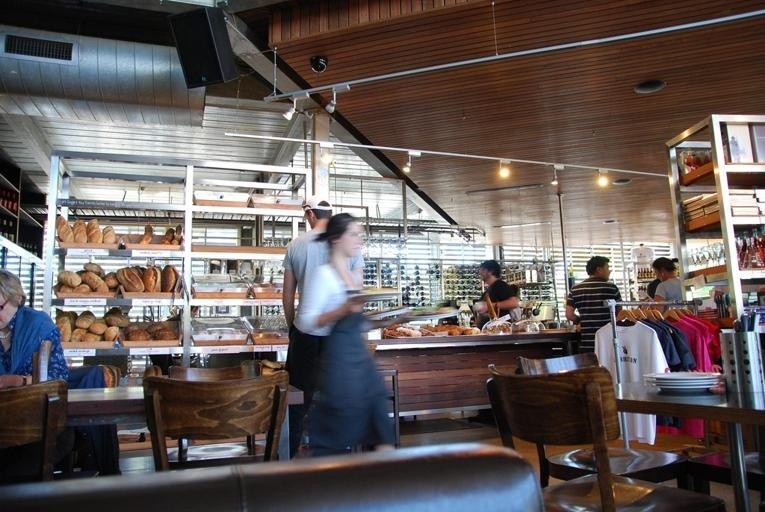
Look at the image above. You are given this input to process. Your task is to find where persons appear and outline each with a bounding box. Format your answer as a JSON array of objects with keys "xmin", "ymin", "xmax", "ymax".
[
  {"xmin": 0, "ymin": 270, "xmax": 76, "ymax": 478},
  {"xmin": 651, "ymin": 257, "xmax": 684, "ymax": 314},
  {"xmin": 263, "ymin": 197, "xmax": 365, "ymax": 461},
  {"xmin": 566, "ymin": 256, "xmax": 622, "ymax": 354},
  {"xmin": 473, "ymin": 259, "xmax": 520, "ymax": 331},
  {"xmin": 293, "ymin": 213, "xmax": 413, "ymax": 458},
  {"xmin": 507, "ymin": 284, "xmax": 521, "ymax": 323}
]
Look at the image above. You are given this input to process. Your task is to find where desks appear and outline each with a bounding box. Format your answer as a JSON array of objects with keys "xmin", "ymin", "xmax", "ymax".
[{"xmin": 69, "ymin": 383, "xmax": 304, "ymax": 461}]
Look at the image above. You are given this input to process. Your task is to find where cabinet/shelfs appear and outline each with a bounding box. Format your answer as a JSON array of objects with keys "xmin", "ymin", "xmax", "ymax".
[
  {"xmin": 1, "ymin": 144, "xmax": 48, "ymax": 264},
  {"xmin": 666, "ymin": 113, "xmax": 764, "ymax": 331},
  {"xmin": 41, "ymin": 148, "xmax": 311, "ymax": 388},
  {"xmin": 363, "ymin": 253, "xmax": 679, "ymax": 322}
]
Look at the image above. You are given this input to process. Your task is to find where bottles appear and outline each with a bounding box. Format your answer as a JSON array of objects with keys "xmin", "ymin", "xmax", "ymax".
[
  {"xmin": 1, "ymin": 218, "xmax": 8, "ymax": 238},
  {"xmin": 638, "ymin": 287, "xmax": 651, "ymax": 302},
  {"xmin": 19, "ymin": 241, "xmax": 39, "ymax": 258},
  {"xmin": 8, "ymin": 220, "xmax": 16, "ymax": 241},
  {"xmin": 737, "ymin": 225, "xmax": 765, "ymax": 271},
  {"xmin": 638, "ymin": 268, "xmax": 658, "ymax": 279},
  {"xmin": 1, "ymin": 191, "xmax": 19, "ymax": 214}
]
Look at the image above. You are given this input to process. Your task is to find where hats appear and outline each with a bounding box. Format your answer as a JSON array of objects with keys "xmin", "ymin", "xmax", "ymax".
[{"xmin": 302, "ymin": 196, "xmax": 333, "ymax": 220}]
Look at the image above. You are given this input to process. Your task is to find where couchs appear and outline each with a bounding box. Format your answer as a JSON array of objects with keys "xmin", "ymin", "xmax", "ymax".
[{"xmin": 0, "ymin": 442, "xmax": 543, "ymax": 512}]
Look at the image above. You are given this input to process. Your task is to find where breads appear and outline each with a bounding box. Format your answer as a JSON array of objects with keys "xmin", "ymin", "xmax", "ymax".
[
  {"xmin": 105, "ymin": 307, "xmax": 129, "ymax": 328},
  {"xmin": 118, "ymin": 267, "xmax": 144, "ymax": 292},
  {"xmin": 87, "ymin": 218, "xmax": 102, "ymax": 243},
  {"xmin": 59, "ymin": 312, "xmax": 78, "ymax": 329},
  {"xmin": 57, "ymin": 316, "xmax": 72, "ymax": 342},
  {"xmin": 162, "ymin": 265, "xmax": 182, "ymax": 294},
  {"xmin": 82, "ymin": 271, "xmax": 109, "ymax": 292},
  {"xmin": 146, "ymin": 323, "xmax": 168, "ymax": 334},
  {"xmin": 512, "ymin": 319, "xmax": 545, "ymax": 333},
  {"xmin": 175, "ymin": 225, "xmax": 181, "ymax": 246},
  {"xmin": 145, "ymin": 265, "xmax": 162, "ymax": 292},
  {"xmin": 128, "ymin": 329, "xmax": 150, "ymax": 340},
  {"xmin": 73, "ymin": 284, "xmax": 91, "ymax": 292},
  {"xmin": 89, "ymin": 322, "xmax": 107, "ymax": 335},
  {"xmin": 76, "ymin": 310, "xmax": 95, "ymax": 329},
  {"xmin": 171, "ymin": 239, "xmax": 179, "ymax": 245},
  {"xmin": 154, "ymin": 329, "xmax": 177, "ymax": 341},
  {"xmin": 138, "ymin": 224, "xmax": 154, "ymax": 246},
  {"xmin": 386, "ymin": 324, "xmax": 481, "ymax": 337},
  {"xmin": 105, "ymin": 272, "xmax": 118, "ymax": 288},
  {"xmin": 161, "ymin": 228, "xmax": 174, "ymax": 245},
  {"xmin": 59, "ymin": 284, "xmax": 72, "ymax": 292},
  {"xmin": 82, "ymin": 333, "xmax": 101, "ymax": 342},
  {"xmin": 56, "ymin": 216, "xmax": 75, "ymax": 246},
  {"xmin": 59, "ymin": 271, "xmax": 82, "ymax": 288},
  {"xmin": 74, "ymin": 220, "xmax": 88, "ymax": 243},
  {"xmin": 71, "ymin": 329, "xmax": 87, "ymax": 342},
  {"xmin": 104, "ymin": 325, "xmax": 119, "ymax": 341},
  {"xmin": 482, "ymin": 321, "xmax": 511, "ymax": 336},
  {"xmin": 103, "ymin": 225, "xmax": 115, "ymax": 244},
  {"xmin": 124, "ymin": 324, "xmax": 143, "ymax": 336},
  {"xmin": 85, "ymin": 263, "xmax": 105, "ymax": 278},
  {"xmin": 135, "ymin": 266, "xmax": 147, "ymax": 278}
]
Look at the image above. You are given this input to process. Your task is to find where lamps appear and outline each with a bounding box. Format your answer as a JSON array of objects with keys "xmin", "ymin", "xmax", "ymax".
[
  {"xmin": 403, "ymin": 159, "xmax": 412, "ymax": 173},
  {"xmin": 282, "ymin": 100, "xmax": 297, "ymax": 121},
  {"xmin": 321, "ymin": 149, "xmax": 332, "ymax": 163},
  {"xmin": 497, "ymin": 164, "xmax": 507, "ymax": 178},
  {"xmin": 325, "ymin": 92, "xmax": 337, "ymax": 114},
  {"xmin": 596, "ymin": 174, "xmax": 609, "ymax": 187}
]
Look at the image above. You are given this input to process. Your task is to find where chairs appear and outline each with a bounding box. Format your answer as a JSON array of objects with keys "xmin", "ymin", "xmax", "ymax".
[
  {"xmin": 514, "ymin": 367, "xmax": 681, "ymax": 488},
  {"xmin": 142, "ymin": 370, "xmax": 289, "ymax": 470},
  {"xmin": 486, "ymin": 365, "xmax": 724, "ymax": 511},
  {"xmin": 66, "ymin": 363, "xmax": 118, "ymax": 387},
  {"xmin": 0, "ymin": 378, "xmax": 69, "ymax": 486},
  {"xmin": 162, "ymin": 364, "xmax": 246, "ymax": 384},
  {"xmin": 378, "ymin": 366, "xmax": 400, "ymax": 446}
]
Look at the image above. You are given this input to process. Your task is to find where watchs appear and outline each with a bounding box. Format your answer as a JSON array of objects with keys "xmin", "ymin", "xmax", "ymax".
[{"xmin": 21, "ymin": 376, "xmax": 28, "ymax": 386}]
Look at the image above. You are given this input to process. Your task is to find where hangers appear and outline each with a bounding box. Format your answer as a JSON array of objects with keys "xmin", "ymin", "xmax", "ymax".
[{"xmin": 605, "ymin": 301, "xmax": 704, "ymax": 324}]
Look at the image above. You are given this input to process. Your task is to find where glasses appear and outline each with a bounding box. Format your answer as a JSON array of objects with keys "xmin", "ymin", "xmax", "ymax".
[{"xmin": 0, "ymin": 295, "xmax": 12, "ymax": 310}]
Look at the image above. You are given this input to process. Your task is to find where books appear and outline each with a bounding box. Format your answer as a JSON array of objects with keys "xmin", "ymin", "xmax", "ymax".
[
  {"xmin": 681, "ymin": 188, "xmax": 765, "ymax": 223},
  {"xmin": 724, "ymin": 124, "xmax": 754, "ymax": 164},
  {"xmin": 750, "ymin": 124, "xmax": 765, "ymax": 165}
]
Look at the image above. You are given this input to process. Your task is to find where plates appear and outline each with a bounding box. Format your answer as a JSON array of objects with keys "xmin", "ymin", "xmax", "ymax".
[
  {"xmin": 643, "ymin": 371, "xmax": 721, "ymax": 392},
  {"xmin": 396, "ymin": 310, "xmax": 465, "ymax": 320},
  {"xmin": 351, "ymin": 291, "xmax": 405, "ymax": 302},
  {"xmin": 364, "ymin": 306, "xmax": 417, "ymax": 320}
]
